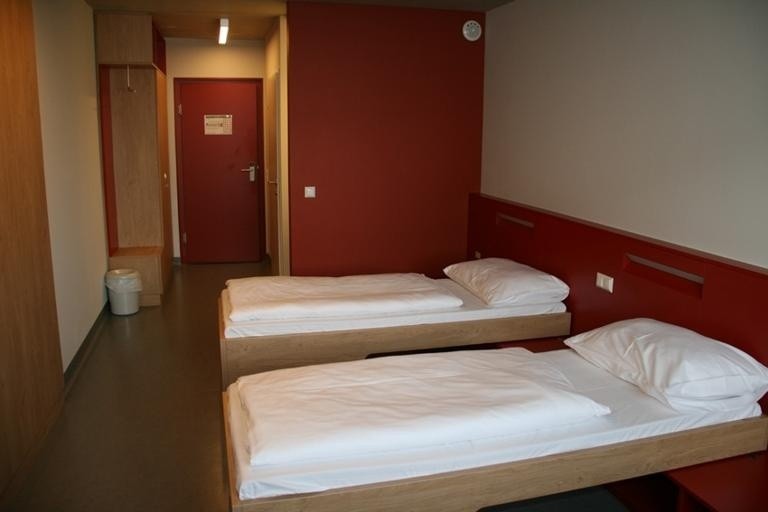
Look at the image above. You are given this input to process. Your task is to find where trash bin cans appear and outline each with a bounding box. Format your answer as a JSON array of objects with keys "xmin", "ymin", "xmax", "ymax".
[{"xmin": 106, "ymin": 267, "xmax": 140, "ymax": 315}]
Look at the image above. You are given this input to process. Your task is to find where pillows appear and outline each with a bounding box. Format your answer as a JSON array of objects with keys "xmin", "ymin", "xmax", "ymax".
[
  {"xmin": 560, "ymin": 317, "xmax": 768, "ymax": 412},
  {"xmin": 442, "ymin": 257, "xmax": 571, "ymax": 307}
]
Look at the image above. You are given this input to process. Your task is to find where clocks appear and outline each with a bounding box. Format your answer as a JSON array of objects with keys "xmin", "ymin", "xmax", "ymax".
[{"xmin": 461, "ymin": 20, "xmax": 482, "ymax": 42}]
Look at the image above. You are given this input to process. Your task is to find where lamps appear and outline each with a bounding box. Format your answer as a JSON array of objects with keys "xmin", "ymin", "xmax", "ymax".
[{"xmin": 217, "ymin": 17, "xmax": 230, "ymax": 46}]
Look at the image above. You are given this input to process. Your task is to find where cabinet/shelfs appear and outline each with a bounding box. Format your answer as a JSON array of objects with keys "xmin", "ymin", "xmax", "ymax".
[{"xmin": 94, "ymin": 9, "xmax": 175, "ymax": 308}]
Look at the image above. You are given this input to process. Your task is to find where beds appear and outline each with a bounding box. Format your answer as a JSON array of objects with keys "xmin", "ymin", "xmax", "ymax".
[
  {"xmin": 218, "ymin": 259, "xmax": 574, "ymax": 388},
  {"xmin": 221, "ymin": 317, "xmax": 768, "ymax": 511}
]
